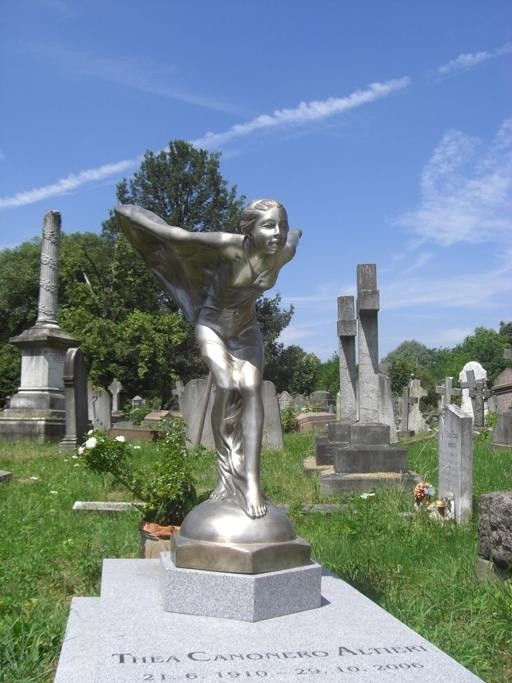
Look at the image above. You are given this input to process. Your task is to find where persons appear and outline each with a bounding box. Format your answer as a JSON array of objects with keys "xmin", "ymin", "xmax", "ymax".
[{"xmin": 113, "ymin": 200, "xmax": 305, "ymax": 519}]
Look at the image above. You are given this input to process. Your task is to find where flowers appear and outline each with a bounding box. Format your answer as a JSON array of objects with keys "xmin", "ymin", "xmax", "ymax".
[
  {"xmin": 65, "ymin": 417, "xmax": 212, "ymax": 520},
  {"xmin": 415, "ymin": 481, "xmax": 436, "ymax": 505}
]
